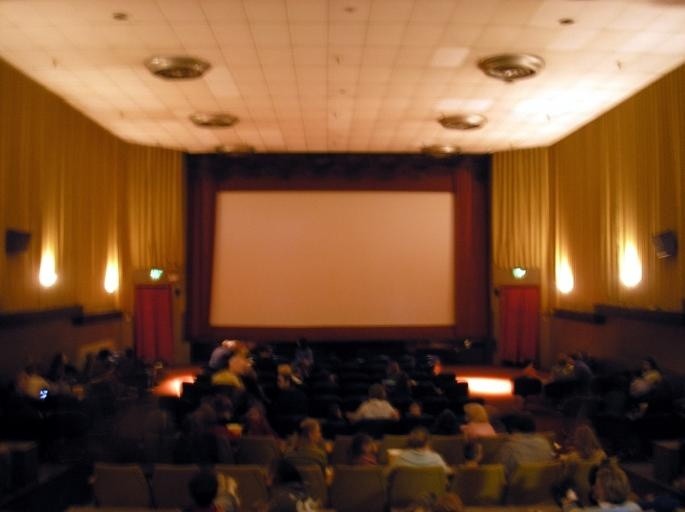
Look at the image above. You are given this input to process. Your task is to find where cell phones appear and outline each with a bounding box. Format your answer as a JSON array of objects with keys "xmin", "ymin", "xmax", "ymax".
[{"xmin": 38, "ymin": 388, "xmax": 50, "ymax": 401}]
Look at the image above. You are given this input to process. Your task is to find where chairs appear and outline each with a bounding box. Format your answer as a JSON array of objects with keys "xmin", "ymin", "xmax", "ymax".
[{"xmin": 0, "ymin": 342, "xmax": 684, "ymax": 511}]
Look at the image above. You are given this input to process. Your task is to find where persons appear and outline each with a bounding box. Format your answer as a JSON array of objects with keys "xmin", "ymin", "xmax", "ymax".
[{"xmin": 1, "ymin": 320, "xmax": 685, "ymax": 512}]
